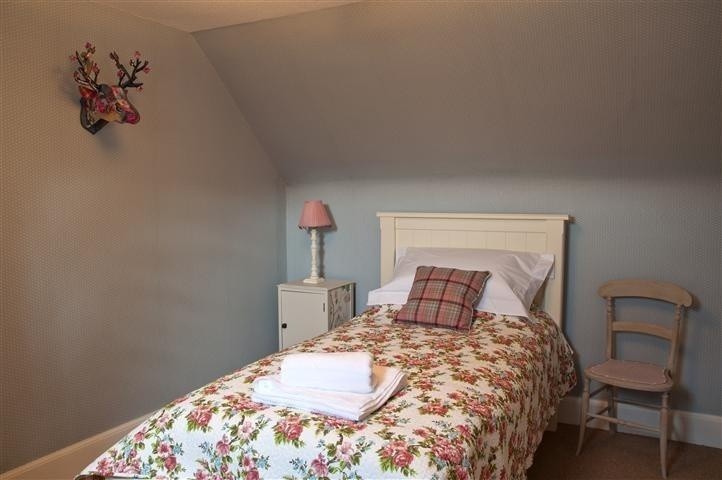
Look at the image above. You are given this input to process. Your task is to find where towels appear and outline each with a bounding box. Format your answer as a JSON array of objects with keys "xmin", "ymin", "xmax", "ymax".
[{"xmin": 252, "ymin": 351, "xmax": 409, "ymax": 423}]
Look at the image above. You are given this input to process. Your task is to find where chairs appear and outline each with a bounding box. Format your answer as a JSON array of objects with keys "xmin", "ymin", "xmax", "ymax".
[{"xmin": 575, "ymin": 275, "xmax": 695, "ymax": 478}]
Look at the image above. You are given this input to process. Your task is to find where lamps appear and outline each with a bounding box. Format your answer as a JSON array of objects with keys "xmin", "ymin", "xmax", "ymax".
[{"xmin": 296, "ymin": 197, "xmax": 334, "ymax": 286}]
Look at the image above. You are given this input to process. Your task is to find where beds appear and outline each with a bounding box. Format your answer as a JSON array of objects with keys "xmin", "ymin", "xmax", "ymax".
[{"xmin": 107, "ymin": 211, "xmax": 573, "ymax": 477}]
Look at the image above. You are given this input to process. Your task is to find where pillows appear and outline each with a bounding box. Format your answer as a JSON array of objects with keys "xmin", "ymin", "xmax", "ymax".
[{"xmin": 367, "ymin": 241, "xmax": 554, "ymax": 334}]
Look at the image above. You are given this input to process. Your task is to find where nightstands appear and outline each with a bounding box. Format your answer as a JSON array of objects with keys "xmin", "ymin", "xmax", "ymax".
[{"xmin": 276, "ymin": 279, "xmax": 356, "ymax": 350}]
